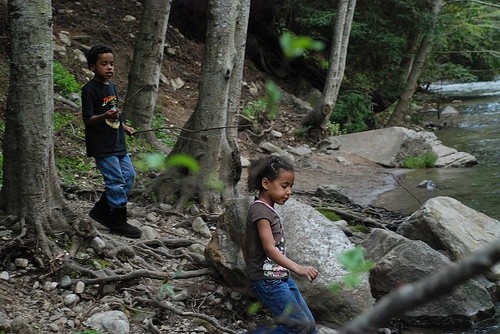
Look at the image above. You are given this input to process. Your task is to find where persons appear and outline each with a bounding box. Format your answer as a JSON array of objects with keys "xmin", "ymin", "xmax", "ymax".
[
  {"xmin": 81, "ymin": 45, "xmax": 142, "ymax": 238},
  {"xmin": 242, "ymin": 155, "xmax": 321, "ymax": 334}
]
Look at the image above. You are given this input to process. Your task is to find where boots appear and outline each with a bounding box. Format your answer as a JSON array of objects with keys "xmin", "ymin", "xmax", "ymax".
[
  {"xmin": 108, "ymin": 207, "xmax": 142, "ymax": 238},
  {"xmin": 89, "ymin": 191, "xmax": 112, "ymax": 228}
]
